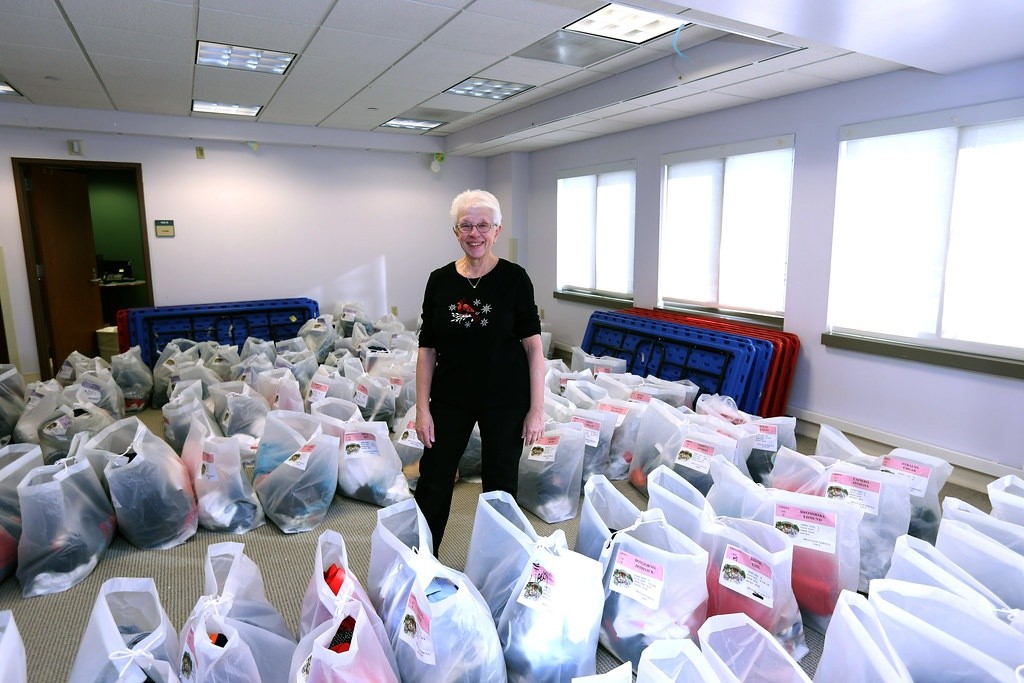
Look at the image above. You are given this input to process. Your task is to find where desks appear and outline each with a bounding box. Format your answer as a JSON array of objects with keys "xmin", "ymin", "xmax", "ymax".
[{"xmin": 98, "ymin": 280, "xmax": 146, "ymax": 323}]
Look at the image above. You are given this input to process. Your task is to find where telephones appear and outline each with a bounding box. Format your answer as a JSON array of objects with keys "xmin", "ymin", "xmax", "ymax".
[{"xmin": 102, "ymin": 269, "xmax": 122, "ymax": 283}]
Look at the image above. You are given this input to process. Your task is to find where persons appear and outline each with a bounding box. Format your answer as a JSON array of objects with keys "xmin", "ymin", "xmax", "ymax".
[{"xmin": 415, "ymin": 188, "xmax": 546, "ymax": 560}]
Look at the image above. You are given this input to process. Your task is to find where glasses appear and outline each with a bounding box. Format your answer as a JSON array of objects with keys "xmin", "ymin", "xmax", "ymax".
[{"xmin": 456, "ymin": 221, "xmax": 497, "ymax": 234}]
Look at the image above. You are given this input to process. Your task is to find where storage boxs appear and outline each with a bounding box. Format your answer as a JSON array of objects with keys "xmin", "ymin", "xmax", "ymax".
[{"xmin": 95, "ymin": 326, "xmax": 118, "ymax": 363}]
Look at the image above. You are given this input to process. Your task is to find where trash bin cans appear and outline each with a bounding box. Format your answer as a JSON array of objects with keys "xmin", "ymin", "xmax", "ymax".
[{"xmin": 96, "ymin": 325, "xmax": 119, "ymax": 363}]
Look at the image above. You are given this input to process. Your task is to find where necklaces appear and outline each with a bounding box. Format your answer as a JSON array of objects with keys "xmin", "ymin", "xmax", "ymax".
[{"xmin": 460, "ymin": 258, "xmax": 490, "ymax": 288}]
